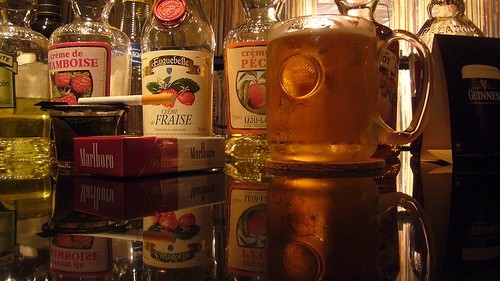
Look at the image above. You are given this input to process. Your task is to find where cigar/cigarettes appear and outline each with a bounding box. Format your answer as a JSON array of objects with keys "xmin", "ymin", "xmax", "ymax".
[
  {"xmin": 70, "ymin": 231, "xmax": 176, "ymax": 244},
  {"xmin": 78, "ymin": 93, "xmax": 175, "ymax": 105}
]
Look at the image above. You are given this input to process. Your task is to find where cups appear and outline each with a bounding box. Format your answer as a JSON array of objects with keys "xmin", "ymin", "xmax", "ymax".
[
  {"xmin": 257, "ymin": 159, "xmax": 431, "ymax": 281},
  {"xmin": 263, "ymin": 15, "xmax": 433, "ymax": 161}
]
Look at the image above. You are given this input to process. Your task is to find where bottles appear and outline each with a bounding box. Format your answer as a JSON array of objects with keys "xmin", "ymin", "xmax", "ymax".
[
  {"xmin": 0, "ymin": 0, "xmax": 289, "ymax": 160},
  {"xmin": 334, "ymin": 0, "xmax": 485, "ymax": 174},
  {"xmin": 0, "ymin": 159, "xmax": 269, "ymax": 281}
]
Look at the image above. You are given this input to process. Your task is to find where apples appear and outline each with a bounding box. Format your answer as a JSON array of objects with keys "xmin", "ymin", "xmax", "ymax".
[
  {"xmin": 247, "ymin": 83, "xmax": 266, "ymax": 109},
  {"xmin": 249, "ymin": 212, "xmax": 267, "ymax": 235}
]
